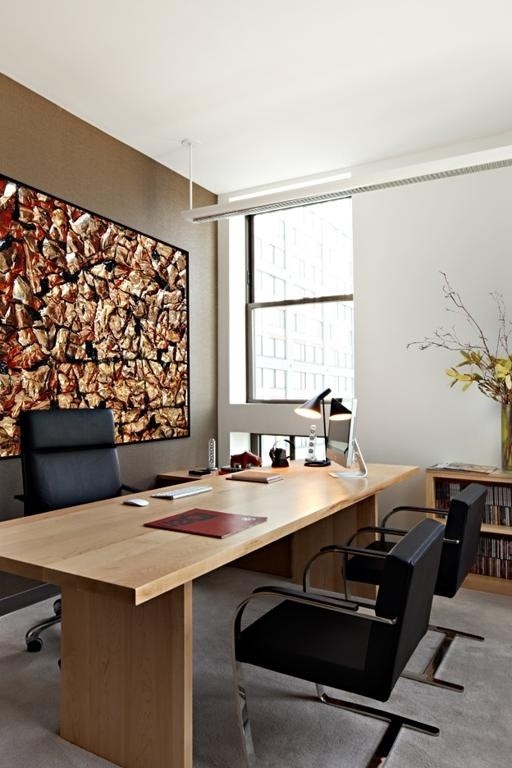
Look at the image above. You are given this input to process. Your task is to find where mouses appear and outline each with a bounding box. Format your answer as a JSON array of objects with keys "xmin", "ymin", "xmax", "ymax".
[{"xmin": 123, "ymin": 498, "xmax": 149, "ymax": 507}]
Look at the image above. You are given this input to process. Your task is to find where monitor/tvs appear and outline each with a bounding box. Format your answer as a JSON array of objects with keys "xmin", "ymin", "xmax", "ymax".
[{"xmin": 326, "ymin": 397, "xmax": 368, "ymax": 478}]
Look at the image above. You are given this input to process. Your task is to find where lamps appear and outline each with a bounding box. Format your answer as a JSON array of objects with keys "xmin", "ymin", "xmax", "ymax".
[
  {"xmin": 329, "ymin": 399, "xmax": 351, "ymax": 422},
  {"xmin": 293, "ymin": 383, "xmax": 334, "ymax": 468},
  {"xmin": 176, "ymin": 131, "xmax": 511, "ymax": 229}
]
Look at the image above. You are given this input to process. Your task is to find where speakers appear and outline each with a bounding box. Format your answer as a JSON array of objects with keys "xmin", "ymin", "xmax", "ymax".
[
  {"xmin": 305, "ymin": 424, "xmax": 317, "ymax": 462},
  {"xmin": 206, "ymin": 437, "xmax": 218, "ymax": 471}
]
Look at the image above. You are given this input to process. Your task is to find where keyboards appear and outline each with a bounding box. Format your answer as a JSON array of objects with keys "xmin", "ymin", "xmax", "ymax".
[{"xmin": 151, "ymin": 487, "xmax": 213, "ymax": 499}]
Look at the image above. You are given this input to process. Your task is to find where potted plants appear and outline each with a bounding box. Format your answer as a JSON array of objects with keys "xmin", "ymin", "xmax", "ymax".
[{"xmin": 408, "ymin": 266, "xmax": 511, "ymax": 474}]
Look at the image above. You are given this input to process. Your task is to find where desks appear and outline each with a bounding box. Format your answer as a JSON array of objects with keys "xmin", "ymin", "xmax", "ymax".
[{"xmin": 0, "ymin": 458, "xmax": 421, "ymax": 768}]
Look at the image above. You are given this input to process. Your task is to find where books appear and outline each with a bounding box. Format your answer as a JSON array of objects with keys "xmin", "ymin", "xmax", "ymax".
[
  {"xmin": 150, "ymin": 485, "xmax": 213, "ymax": 499},
  {"xmin": 434, "ymin": 478, "xmax": 512, "ymax": 578},
  {"xmin": 231, "ymin": 468, "xmax": 282, "ymax": 480},
  {"xmin": 225, "ymin": 475, "xmax": 284, "ymax": 485},
  {"xmin": 142, "ymin": 505, "xmax": 268, "ymax": 540}
]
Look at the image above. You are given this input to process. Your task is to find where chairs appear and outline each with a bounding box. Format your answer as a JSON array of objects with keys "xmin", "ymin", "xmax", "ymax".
[
  {"xmin": 341, "ymin": 481, "xmax": 499, "ymax": 694},
  {"xmin": 227, "ymin": 516, "xmax": 454, "ymax": 767},
  {"xmin": 11, "ymin": 402, "xmax": 147, "ymax": 653}
]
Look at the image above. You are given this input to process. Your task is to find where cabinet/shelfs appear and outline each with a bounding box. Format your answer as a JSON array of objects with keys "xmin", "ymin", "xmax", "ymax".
[
  {"xmin": 157, "ymin": 465, "xmax": 219, "ymax": 485},
  {"xmin": 421, "ymin": 462, "xmax": 512, "ymax": 598}
]
